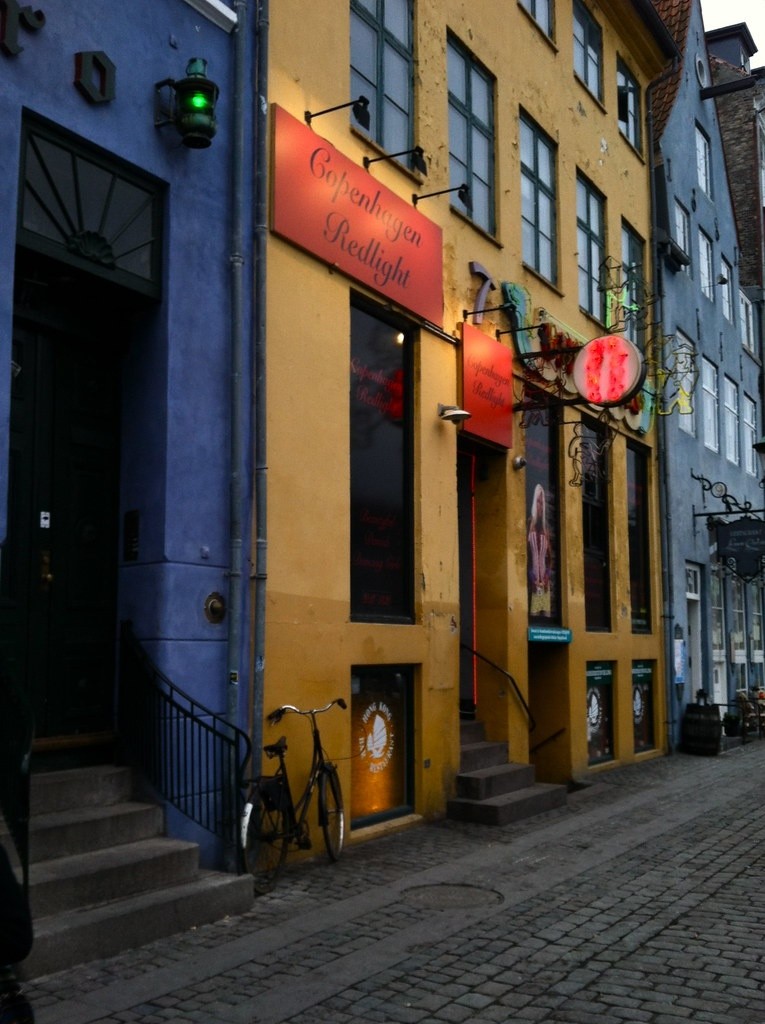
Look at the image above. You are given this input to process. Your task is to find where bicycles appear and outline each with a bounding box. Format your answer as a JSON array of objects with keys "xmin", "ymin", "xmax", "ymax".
[{"xmin": 235, "ymin": 698, "xmax": 349, "ymax": 896}]
